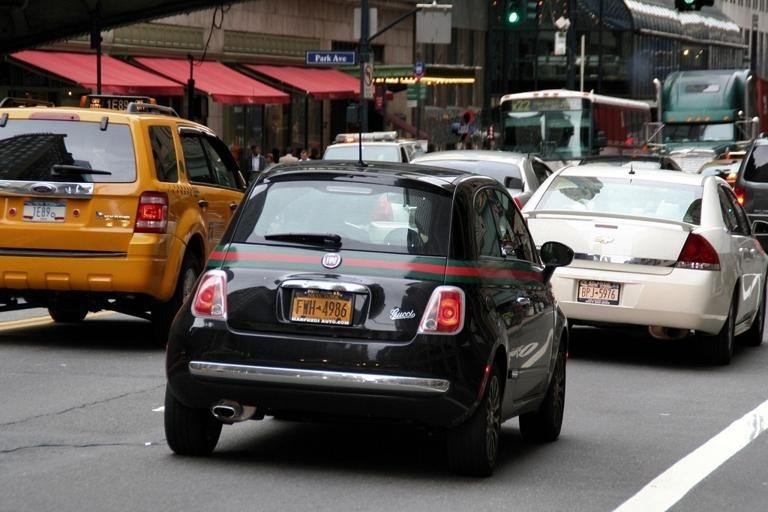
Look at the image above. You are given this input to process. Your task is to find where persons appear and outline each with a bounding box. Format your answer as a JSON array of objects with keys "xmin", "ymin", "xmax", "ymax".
[{"xmin": 242, "ymin": 144, "xmax": 312, "ymax": 186}]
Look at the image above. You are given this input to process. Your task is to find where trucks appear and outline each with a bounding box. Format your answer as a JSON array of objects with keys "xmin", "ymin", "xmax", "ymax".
[{"xmin": 659, "ymin": 66, "xmax": 768, "ymax": 143}]
